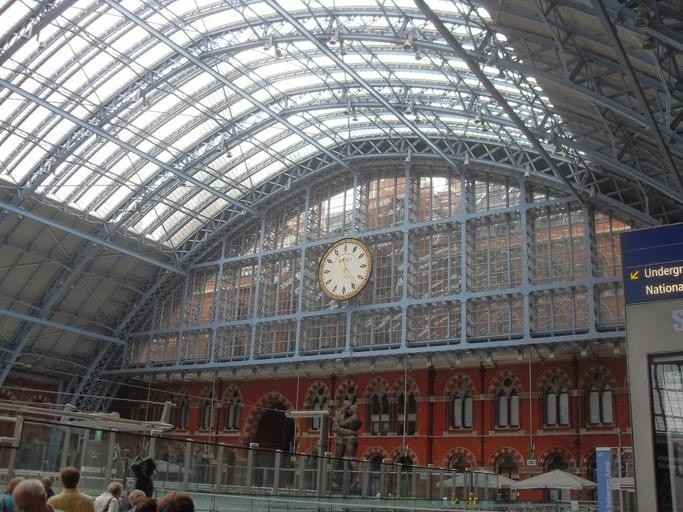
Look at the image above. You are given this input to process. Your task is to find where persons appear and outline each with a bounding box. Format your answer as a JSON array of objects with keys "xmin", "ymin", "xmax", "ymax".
[
  {"xmin": 331, "ymin": 399, "xmax": 356, "ymax": 488},
  {"xmin": 120, "ymin": 448, "xmax": 131, "ymax": 466},
  {"xmin": 335, "ymin": 404, "xmax": 362, "ymax": 487},
  {"xmin": 0, "ymin": 467, "xmax": 195, "ymax": 512},
  {"xmin": 450, "ymin": 492, "xmax": 458, "ymax": 508},
  {"xmin": 465, "ymin": 492, "xmax": 476, "ymax": 511}
]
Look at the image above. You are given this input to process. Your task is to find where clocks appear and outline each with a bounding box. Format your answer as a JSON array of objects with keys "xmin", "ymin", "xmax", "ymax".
[{"xmin": 316, "ymin": 237, "xmax": 374, "ymax": 301}]
